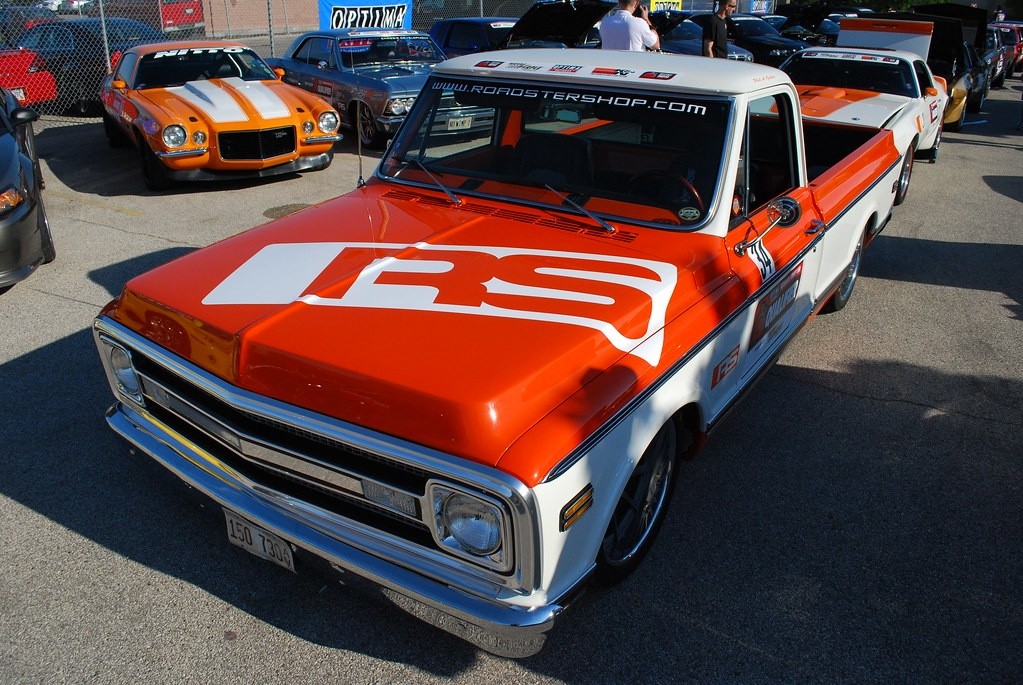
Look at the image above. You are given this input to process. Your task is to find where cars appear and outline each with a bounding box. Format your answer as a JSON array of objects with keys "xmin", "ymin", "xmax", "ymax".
[
  {"xmin": 426, "ymin": 0, "xmax": 619, "ymax": 60},
  {"xmin": 252, "ymin": 29, "xmax": 493, "ymax": 146},
  {"xmin": 746, "ymin": 19, "xmax": 953, "ymax": 202},
  {"xmin": 96, "ymin": 38, "xmax": 345, "ymax": 187},
  {"xmin": 599, "ymin": 5, "xmax": 1023, "ymax": 129},
  {"xmin": 0, "ymin": 86, "xmax": 58, "ymax": 291},
  {"xmin": 0, "ymin": 6, "xmax": 177, "ymax": 116}
]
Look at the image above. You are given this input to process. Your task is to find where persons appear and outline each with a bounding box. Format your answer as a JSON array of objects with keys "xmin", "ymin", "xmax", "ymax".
[
  {"xmin": 599, "ymin": 0, "xmax": 660, "ymax": 52},
  {"xmin": 702, "ymin": 0, "xmax": 737, "ymax": 59}
]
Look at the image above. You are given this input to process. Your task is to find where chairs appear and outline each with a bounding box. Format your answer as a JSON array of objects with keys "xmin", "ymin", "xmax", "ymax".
[{"xmin": 494, "ymin": 133, "xmax": 724, "ymax": 220}]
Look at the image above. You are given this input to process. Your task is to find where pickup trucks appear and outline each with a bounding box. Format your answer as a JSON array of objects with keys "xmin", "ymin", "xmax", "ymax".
[{"xmin": 90, "ymin": 47, "xmax": 905, "ymax": 639}]
